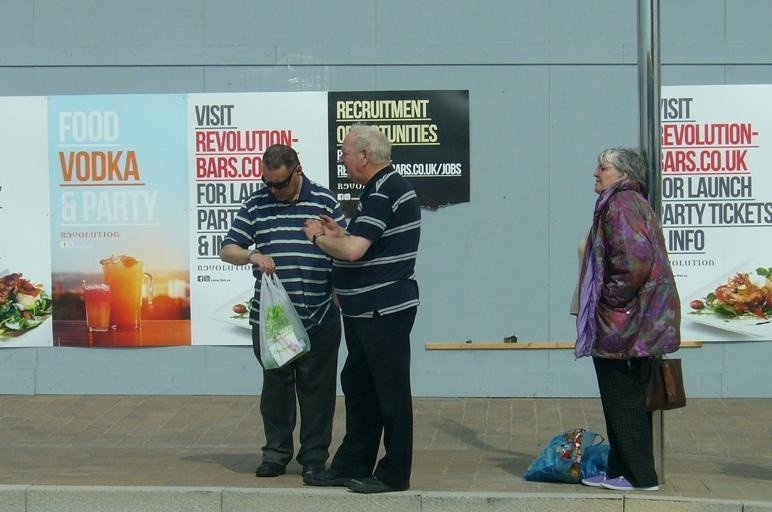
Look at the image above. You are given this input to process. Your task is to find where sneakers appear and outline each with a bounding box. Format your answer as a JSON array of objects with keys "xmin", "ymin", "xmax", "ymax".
[
  {"xmin": 344, "ymin": 474, "xmax": 410, "ymax": 494},
  {"xmin": 600, "ymin": 475, "xmax": 659, "ymax": 491},
  {"xmin": 581, "ymin": 471, "xmax": 611, "ymax": 487}
]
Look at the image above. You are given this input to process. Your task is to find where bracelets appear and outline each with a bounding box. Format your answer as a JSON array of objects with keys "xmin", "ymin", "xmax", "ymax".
[
  {"xmin": 248, "ymin": 250, "xmax": 261, "ymax": 261},
  {"xmin": 313, "ymin": 232, "xmax": 326, "ymax": 246}
]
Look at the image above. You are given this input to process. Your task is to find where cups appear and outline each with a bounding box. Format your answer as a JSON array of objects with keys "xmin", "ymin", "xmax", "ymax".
[
  {"xmin": 84, "ymin": 284, "xmax": 112, "ymax": 334},
  {"xmin": 101, "ymin": 258, "xmax": 155, "ymax": 332}
]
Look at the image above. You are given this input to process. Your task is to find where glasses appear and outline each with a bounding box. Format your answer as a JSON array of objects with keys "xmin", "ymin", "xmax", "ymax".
[
  {"xmin": 594, "ymin": 165, "xmax": 614, "ymax": 173},
  {"xmin": 262, "ymin": 162, "xmax": 300, "ymax": 189}
]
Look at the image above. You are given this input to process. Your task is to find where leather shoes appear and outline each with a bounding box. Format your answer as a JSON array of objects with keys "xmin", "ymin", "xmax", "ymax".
[
  {"xmin": 302, "ymin": 460, "xmax": 326, "ymax": 478},
  {"xmin": 303, "ymin": 468, "xmax": 371, "ymax": 487},
  {"xmin": 256, "ymin": 461, "xmax": 286, "ymax": 478}
]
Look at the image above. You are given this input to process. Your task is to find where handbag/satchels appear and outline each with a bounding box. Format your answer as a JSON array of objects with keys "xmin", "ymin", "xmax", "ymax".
[
  {"xmin": 526, "ymin": 430, "xmax": 612, "ymax": 486},
  {"xmin": 641, "ymin": 358, "xmax": 687, "ymax": 412},
  {"xmin": 258, "ymin": 273, "xmax": 312, "ymax": 368}
]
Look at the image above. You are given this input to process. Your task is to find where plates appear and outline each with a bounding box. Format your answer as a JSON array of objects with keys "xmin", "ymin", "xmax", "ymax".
[
  {"xmin": 209, "ymin": 286, "xmax": 253, "ymax": 328},
  {"xmin": 678, "ymin": 258, "xmax": 772, "ymax": 338}
]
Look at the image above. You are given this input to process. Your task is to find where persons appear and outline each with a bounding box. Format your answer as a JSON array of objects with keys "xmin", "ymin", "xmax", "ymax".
[
  {"xmin": 217, "ymin": 141, "xmax": 348, "ymax": 483},
  {"xmin": 566, "ymin": 146, "xmax": 683, "ymax": 492},
  {"xmin": 303, "ymin": 125, "xmax": 422, "ymax": 495}
]
